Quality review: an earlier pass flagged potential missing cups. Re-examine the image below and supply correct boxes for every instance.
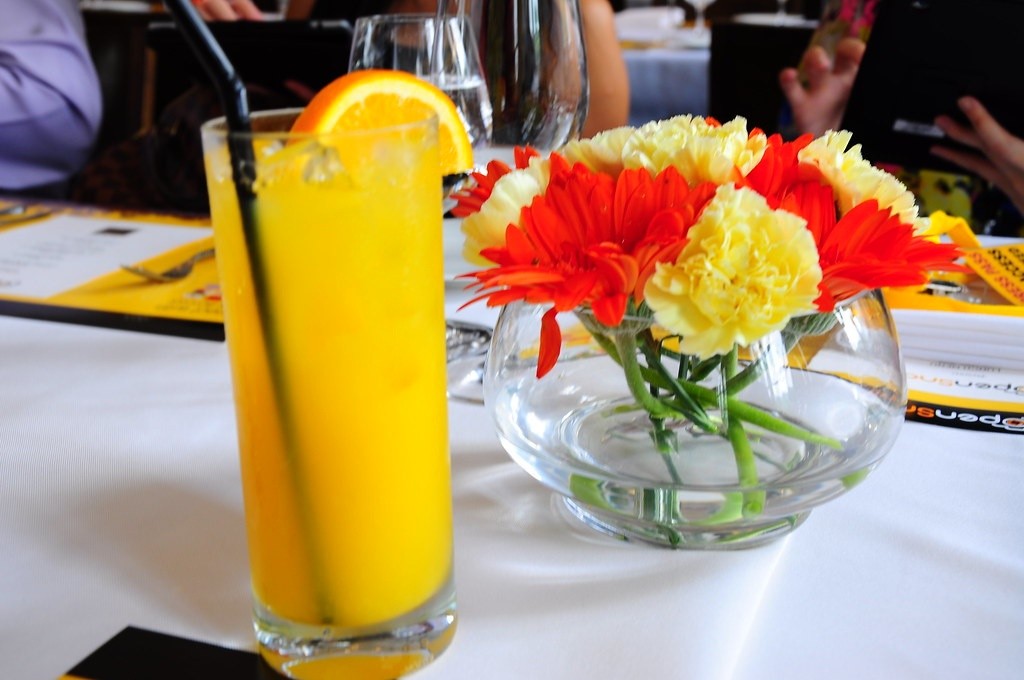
[428,0,588,179]
[346,14,493,181]
[202,107,456,680]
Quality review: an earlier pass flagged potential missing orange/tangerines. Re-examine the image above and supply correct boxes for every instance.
[283,70,474,178]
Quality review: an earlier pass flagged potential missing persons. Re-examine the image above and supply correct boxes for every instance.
[706,0,1024,238]
[191,1,632,146]
[0,0,103,203]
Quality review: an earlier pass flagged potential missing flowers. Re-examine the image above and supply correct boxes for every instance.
[449,111,980,542]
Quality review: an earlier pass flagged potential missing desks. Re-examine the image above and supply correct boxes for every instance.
[0,190,1021,679]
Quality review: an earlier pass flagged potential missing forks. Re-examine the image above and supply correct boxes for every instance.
[121,247,215,282]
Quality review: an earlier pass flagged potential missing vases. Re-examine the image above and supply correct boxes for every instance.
[481,284,907,550]
